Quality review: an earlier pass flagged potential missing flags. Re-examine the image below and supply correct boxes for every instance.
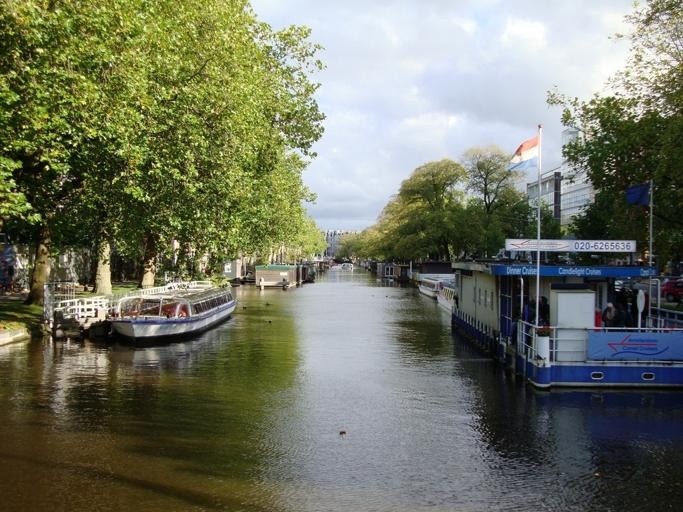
[508,133,540,163]
[625,182,652,209]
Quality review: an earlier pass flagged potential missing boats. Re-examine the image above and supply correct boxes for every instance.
[108,283,240,343]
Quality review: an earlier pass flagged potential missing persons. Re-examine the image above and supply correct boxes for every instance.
[0,250,9,293]
[524,283,649,336]
[4,247,17,292]
